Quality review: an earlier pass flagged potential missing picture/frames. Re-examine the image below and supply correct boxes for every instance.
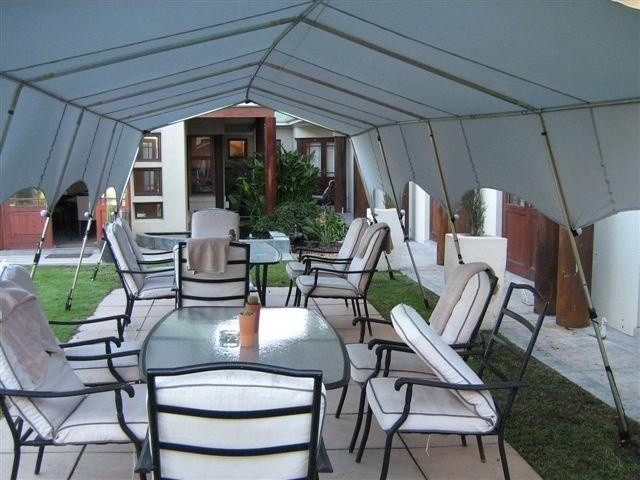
[228,139,247,158]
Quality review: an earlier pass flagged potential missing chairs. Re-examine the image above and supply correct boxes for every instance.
[311,179,335,210]
[133,358,336,479]
[189,208,241,239]
[167,238,250,306]
[0,262,151,480]
[296,221,394,337]
[284,216,373,308]
[101,216,175,325]
[355,279,549,479]
[332,260,499,454]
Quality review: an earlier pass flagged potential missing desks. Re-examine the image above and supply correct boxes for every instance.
[172,237,283,307]
[137,305,353,392]
